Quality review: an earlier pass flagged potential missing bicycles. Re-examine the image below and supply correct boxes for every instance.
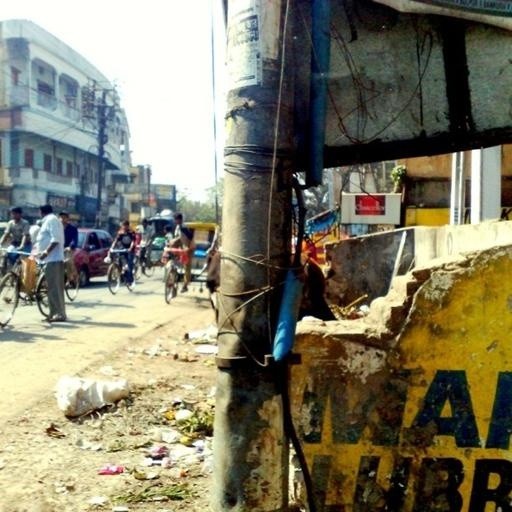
[0,247,80,329]
[108,246,154,293]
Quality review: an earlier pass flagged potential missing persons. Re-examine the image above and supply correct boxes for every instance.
[133,224,142,258]
[28,219,42,244]
[165,212,197,292]
[161,224,174,265]
[28,204,68,323]
[135,219,156,273]
[0,205,32,276]
[109,219,136,287]
[57,210,79,288]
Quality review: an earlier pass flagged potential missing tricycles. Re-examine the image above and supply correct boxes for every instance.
[138,215,176,266]
[160,222,220,302]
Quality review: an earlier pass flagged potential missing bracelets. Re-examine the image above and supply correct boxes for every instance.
[20,245,25,248]
[43,249,49,258]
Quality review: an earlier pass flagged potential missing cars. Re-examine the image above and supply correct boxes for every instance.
[40,228,113,288]
